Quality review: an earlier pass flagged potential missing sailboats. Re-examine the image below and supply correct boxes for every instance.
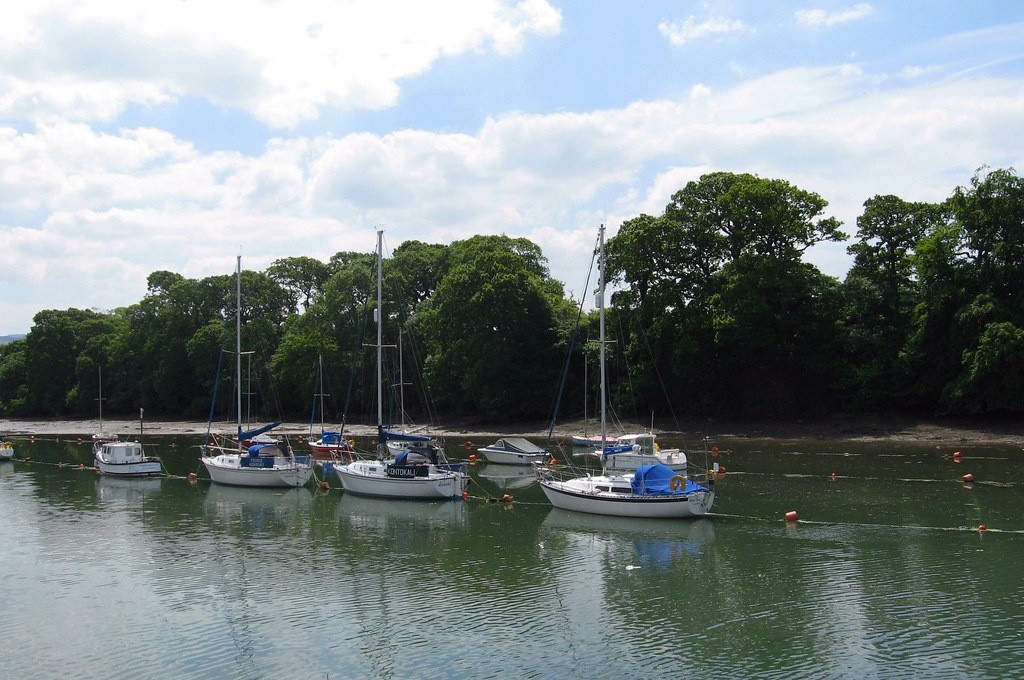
[200,256,314,488]
[329,229,471,501]
[385,326,446,461]
[531,223,725,516]
[307,353,355,460]
[571,349,658,447]
[91,366,119,444]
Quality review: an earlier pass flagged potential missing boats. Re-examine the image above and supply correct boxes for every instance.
[594,412,689,475]
[476,437,553,465]
[0,440,14,460]
[93,439,164,476]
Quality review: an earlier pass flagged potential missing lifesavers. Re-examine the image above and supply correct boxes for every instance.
[4,442,12,450]
[670,474,687,491]
[346,439,357,451]
